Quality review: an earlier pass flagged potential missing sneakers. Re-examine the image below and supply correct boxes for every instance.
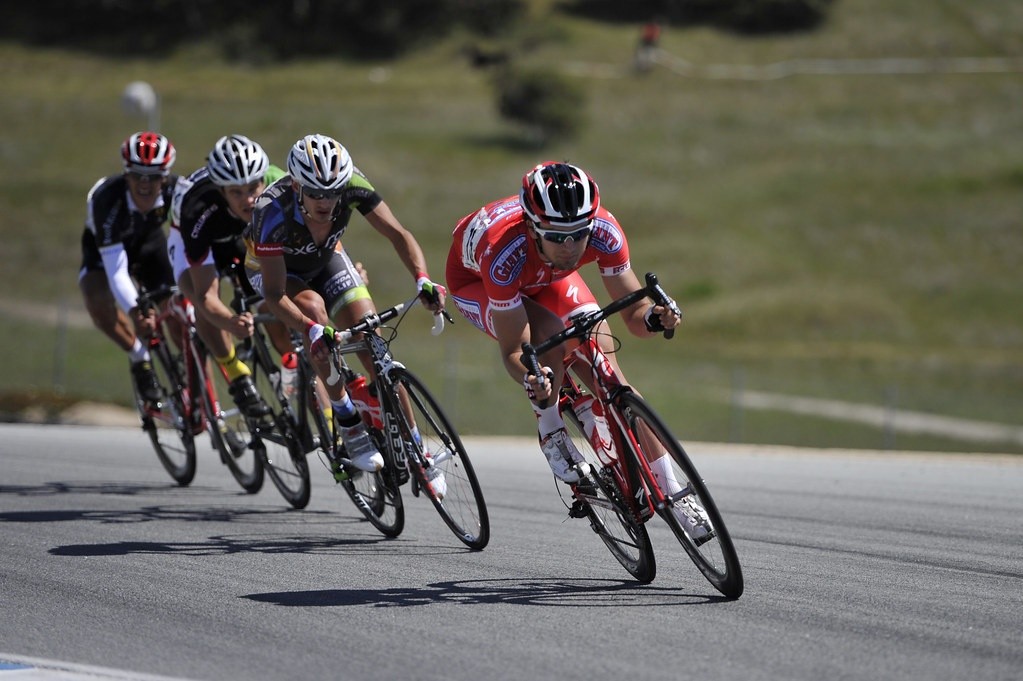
[538,428,591,482]
[415,448,447,498]
[228,375,270,417]
[221,424,243,458]
[128,350,163,401]
[673,495,714,539]
[334,416,384,472]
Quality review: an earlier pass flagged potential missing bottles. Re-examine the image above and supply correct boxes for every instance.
[571,393,617,464]
[368,380,382,430]
[268,365,290,407]
[281,352,297,399]
[591,399,618,462]
[345,368,371,424]
[175,354,188,388]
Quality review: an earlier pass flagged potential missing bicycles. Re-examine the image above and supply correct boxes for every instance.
[228,290,385,518]
[519,271,744,601]
[314,282,490,550]
[126,283,265,493]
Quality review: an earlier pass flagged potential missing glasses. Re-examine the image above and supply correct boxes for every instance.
[220,185,265,200]
[130,172,163,184]
[531,219,594,244]
[302,185,344,200]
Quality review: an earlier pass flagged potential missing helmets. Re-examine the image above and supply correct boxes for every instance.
[519,161,600,226]
[208,134,270,186]
[287,134,353,190]
[121,131,176,176]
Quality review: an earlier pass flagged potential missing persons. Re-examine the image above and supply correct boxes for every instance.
[446,162,717,547]
[244,133,447,499]
[79,130,370,463]
